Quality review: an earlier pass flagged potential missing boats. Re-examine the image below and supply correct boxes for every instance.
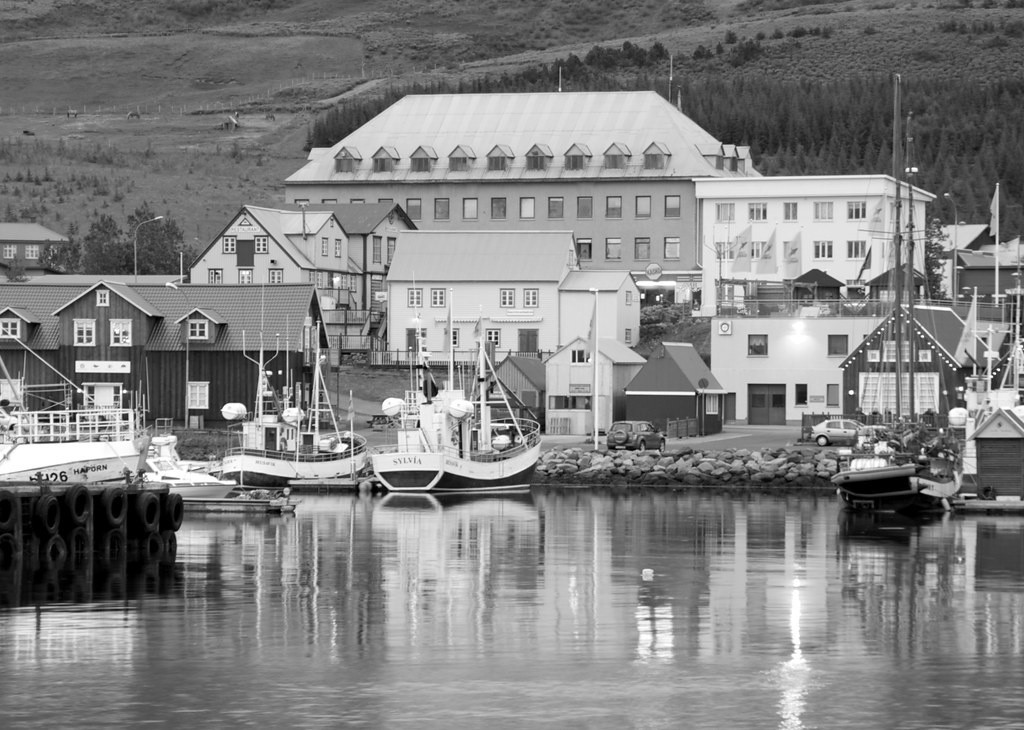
[221,288,368,489]
[141,445,238,504]
[361,289,545,491]
[1,324,144,499]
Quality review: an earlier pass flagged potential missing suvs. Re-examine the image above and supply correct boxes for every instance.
[608,422,670,451]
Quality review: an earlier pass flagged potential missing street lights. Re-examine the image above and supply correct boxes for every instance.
[133,214,165,280]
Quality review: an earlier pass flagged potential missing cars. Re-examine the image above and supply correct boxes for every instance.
[812,419,888,447]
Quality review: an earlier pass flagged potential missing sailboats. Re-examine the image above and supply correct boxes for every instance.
[830,68,965,522]
